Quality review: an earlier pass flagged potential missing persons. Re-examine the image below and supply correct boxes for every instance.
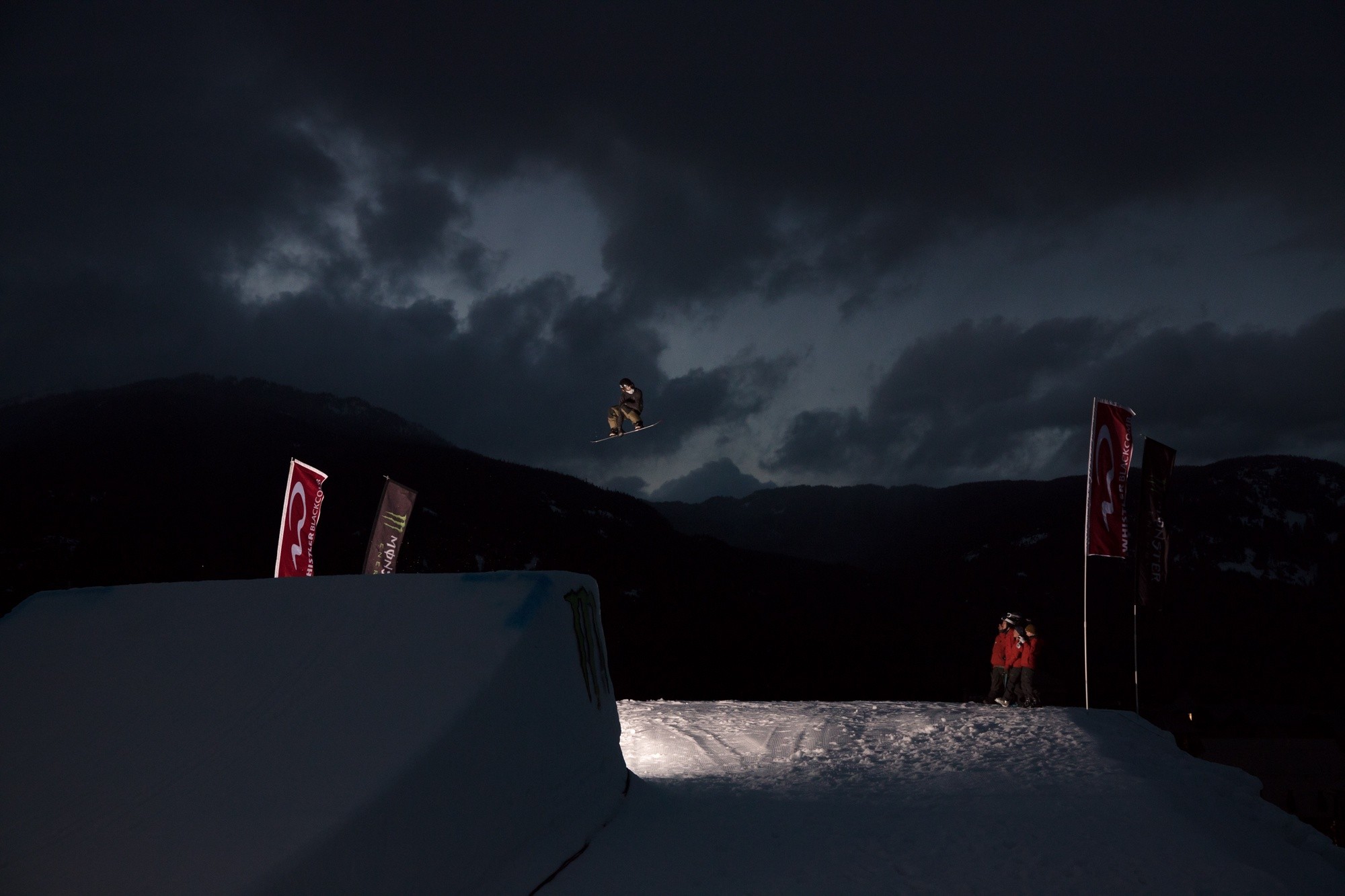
[982,613,1043,708]
[607,378,644,436]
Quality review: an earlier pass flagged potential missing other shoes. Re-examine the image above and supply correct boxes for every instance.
[1028,698,1046,709]
[1023,699,1032,708]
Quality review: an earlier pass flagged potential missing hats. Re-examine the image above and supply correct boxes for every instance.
[1015,625,1024,636]
[1025,624,1037,634]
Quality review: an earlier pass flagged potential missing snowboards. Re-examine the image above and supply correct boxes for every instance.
[590,419,663,444]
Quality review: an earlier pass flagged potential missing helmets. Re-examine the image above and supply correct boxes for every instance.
[1001,613,1021,625]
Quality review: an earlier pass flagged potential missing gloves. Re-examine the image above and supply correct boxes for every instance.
[617,427,625,437]
[618,403,625,411]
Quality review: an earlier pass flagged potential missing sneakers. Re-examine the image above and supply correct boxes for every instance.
[983,697,997,705]
[995,698,1009,707]
[635,421,643,429]
[609,428,618,436]
